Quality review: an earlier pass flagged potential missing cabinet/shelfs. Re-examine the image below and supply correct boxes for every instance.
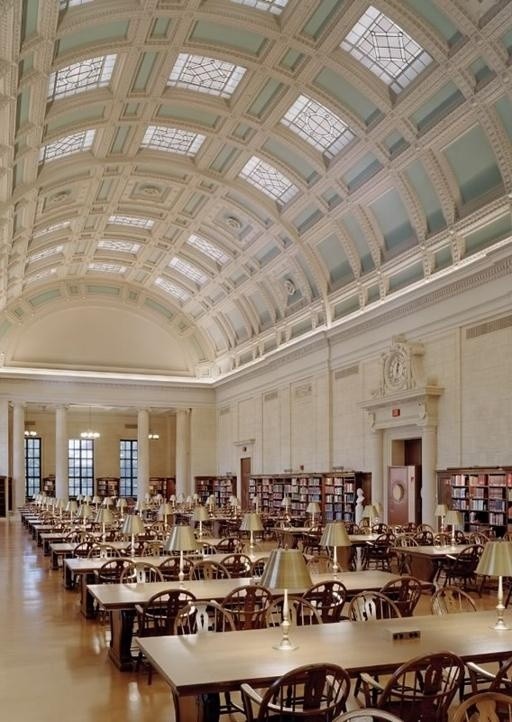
[98,471,370,525]
[436,465,512,536]
[42,474,55,498]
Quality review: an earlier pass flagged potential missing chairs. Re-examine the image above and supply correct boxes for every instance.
[18,494,512,722]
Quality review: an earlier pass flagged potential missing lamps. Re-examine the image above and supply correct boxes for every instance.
[148,414,160,440]
[23,406,36,437]
[79,406,101,439]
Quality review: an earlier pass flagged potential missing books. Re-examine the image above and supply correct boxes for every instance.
[195,478,354,521]
[44,479,55,496]
[451,474,512,526]
[98,480,118,496]
[149,480,167,497]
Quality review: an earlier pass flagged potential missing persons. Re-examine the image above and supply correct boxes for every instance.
[355,488,365,524]
